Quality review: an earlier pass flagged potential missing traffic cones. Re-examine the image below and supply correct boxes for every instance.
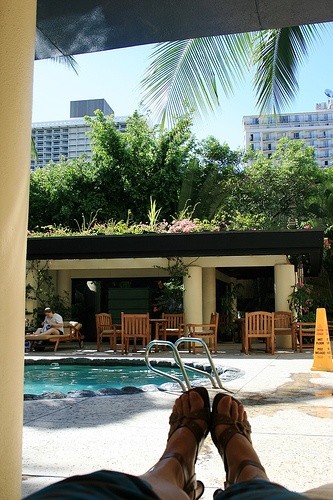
[310,307,333,372]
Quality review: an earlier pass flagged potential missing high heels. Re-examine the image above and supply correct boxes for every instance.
[210,393,265,500]
[160,387,210,500]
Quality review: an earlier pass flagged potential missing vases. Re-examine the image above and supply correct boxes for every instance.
[221,330,231,343]
[297,327,304,344]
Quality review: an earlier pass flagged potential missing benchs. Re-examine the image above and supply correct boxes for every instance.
[296,320,333,352]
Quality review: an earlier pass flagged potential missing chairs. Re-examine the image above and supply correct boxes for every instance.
[122,311,152,355]
[273,311,296,350]
[25,321,85,351]
[187,312,220,355]
[95,313,122,354]
[156,312,187,350]
[245,311,275,354]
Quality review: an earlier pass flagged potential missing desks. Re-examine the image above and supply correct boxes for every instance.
[148,319,175,352]
[232,318,285,352]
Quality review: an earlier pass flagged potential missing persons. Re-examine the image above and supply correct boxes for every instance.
[33,306,65,335]
[22,386,312,499]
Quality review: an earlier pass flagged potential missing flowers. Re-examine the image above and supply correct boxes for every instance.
[221,283,244,332]
[153,256,191,313]
[287,280,314,322]
